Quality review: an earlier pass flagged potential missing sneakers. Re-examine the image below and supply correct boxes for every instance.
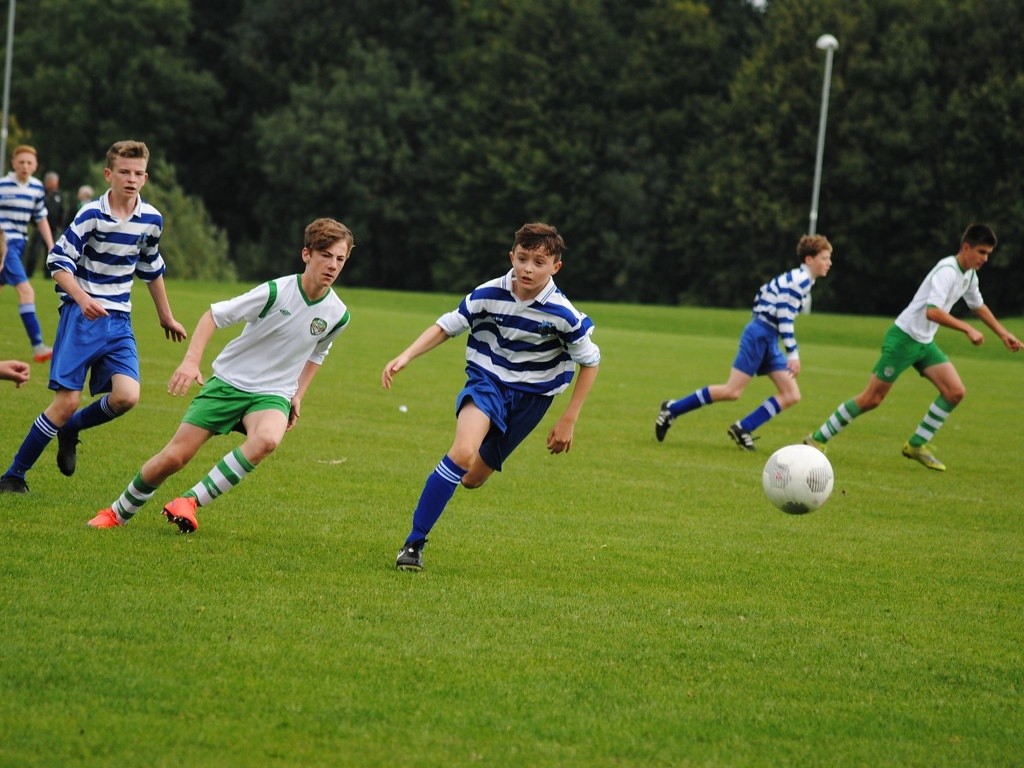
[728,421,757,454]
[394,538,428,572]
[654,398,678,442]
[86,505,123,531]
[57,426,80,477]
[901,439,948,472]
[805,433,828,455]
[158,495,199,534]
[33,345,54,364]
[0,474,30,495]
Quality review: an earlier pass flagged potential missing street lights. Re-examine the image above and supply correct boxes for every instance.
[802,34,840,315]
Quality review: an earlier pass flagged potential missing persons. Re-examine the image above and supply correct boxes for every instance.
[0,139,188,496]
[84,218,357,535]
[66,185,94,230]
[23,171,66,278]
[802,219,1024,471]
[380,219,603,573]
[0,145,54,363]
[0,360,31,389]
[653,234,832,451]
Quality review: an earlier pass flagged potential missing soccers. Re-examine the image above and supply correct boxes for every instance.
[762,443,834,516]
